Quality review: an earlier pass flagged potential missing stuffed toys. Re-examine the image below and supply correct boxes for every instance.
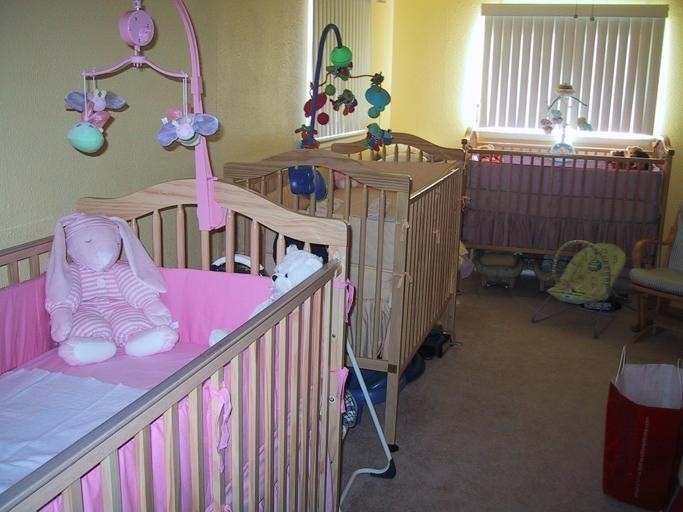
[476,144,504,163]
[63,89,125,112]
[43,212,181,365]
[610,151,624,168]
[67,102,110,154]
[626,146,649,169]
[156,106,218,148]
[209,245,322,347]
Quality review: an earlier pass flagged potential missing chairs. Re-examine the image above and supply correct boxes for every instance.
[530,238,628,340]
[625,203,683,348]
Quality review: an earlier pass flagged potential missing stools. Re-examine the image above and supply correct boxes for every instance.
[475,254,522,289]
[533,259,564,295]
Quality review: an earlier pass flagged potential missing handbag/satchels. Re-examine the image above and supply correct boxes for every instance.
[602,347,683,509]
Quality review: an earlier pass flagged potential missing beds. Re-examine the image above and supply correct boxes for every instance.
[0,176,350,512]
[218,126,466,452]
[458,127,673,297]
